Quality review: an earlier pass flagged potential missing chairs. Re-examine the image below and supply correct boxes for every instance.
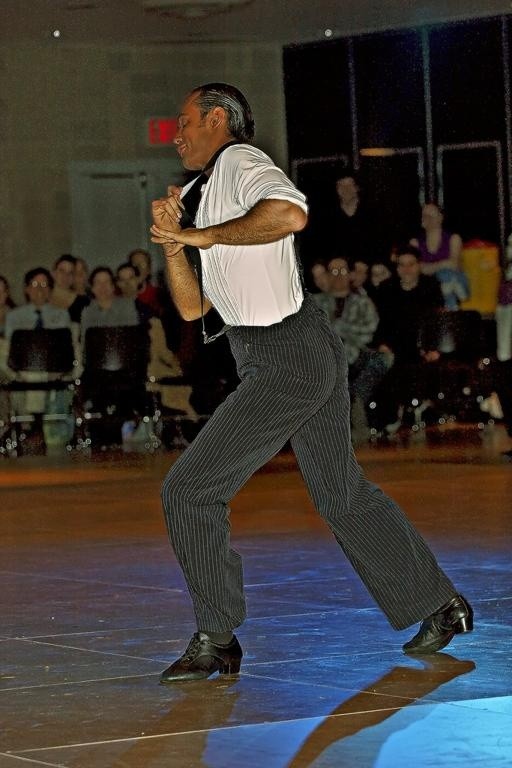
[0,309,492,457]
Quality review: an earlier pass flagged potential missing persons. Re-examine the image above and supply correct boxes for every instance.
[308,247,512,445]
[314,173,384,264]
[0,248,241,461]
[403,203,473,312]
[144,78,478,687]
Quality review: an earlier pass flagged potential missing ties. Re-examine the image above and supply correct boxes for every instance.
[34,309,44,329]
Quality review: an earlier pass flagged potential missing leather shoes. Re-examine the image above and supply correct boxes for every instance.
[402,593,475,655]
[159,631,242,682]
